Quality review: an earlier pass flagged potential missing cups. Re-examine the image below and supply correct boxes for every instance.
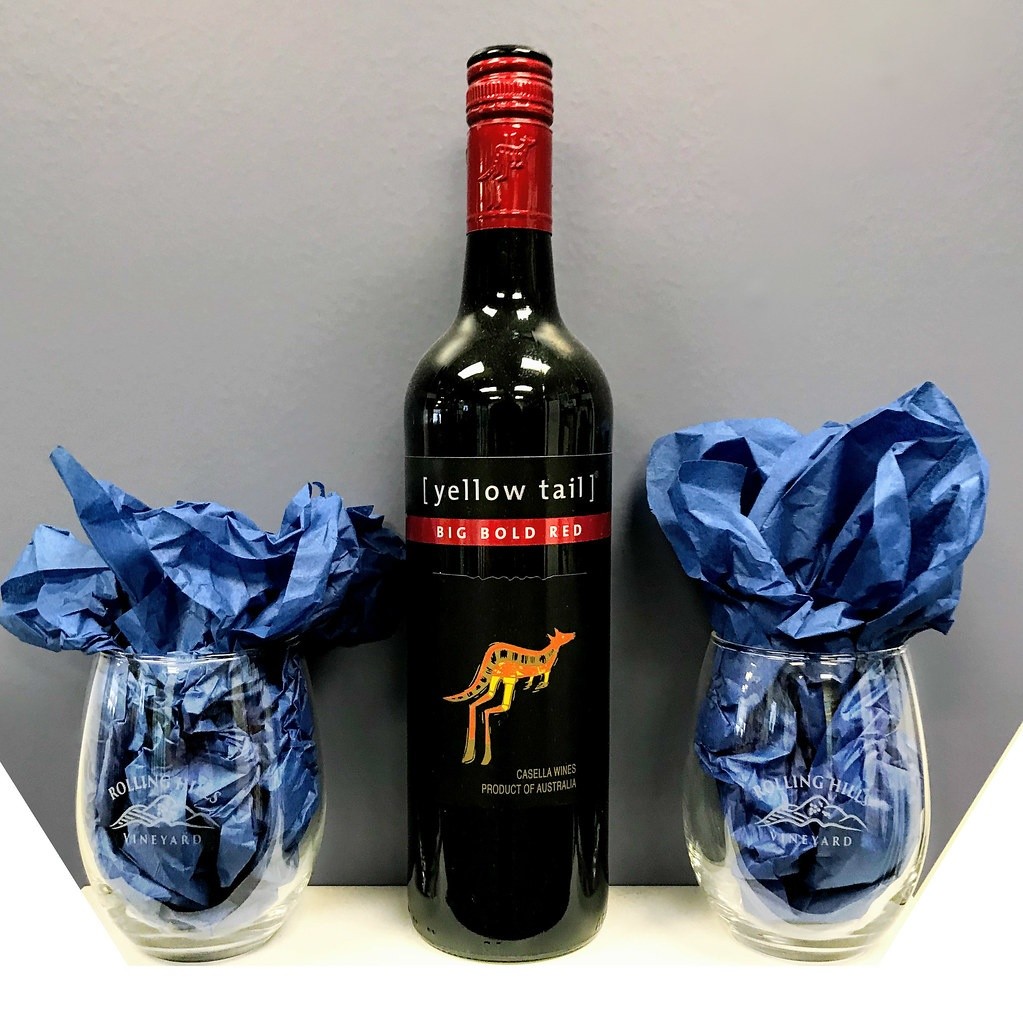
[75,633,328,963]
[681,631,931,961]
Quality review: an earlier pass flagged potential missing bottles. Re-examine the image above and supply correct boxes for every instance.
[404,46,613,964]
